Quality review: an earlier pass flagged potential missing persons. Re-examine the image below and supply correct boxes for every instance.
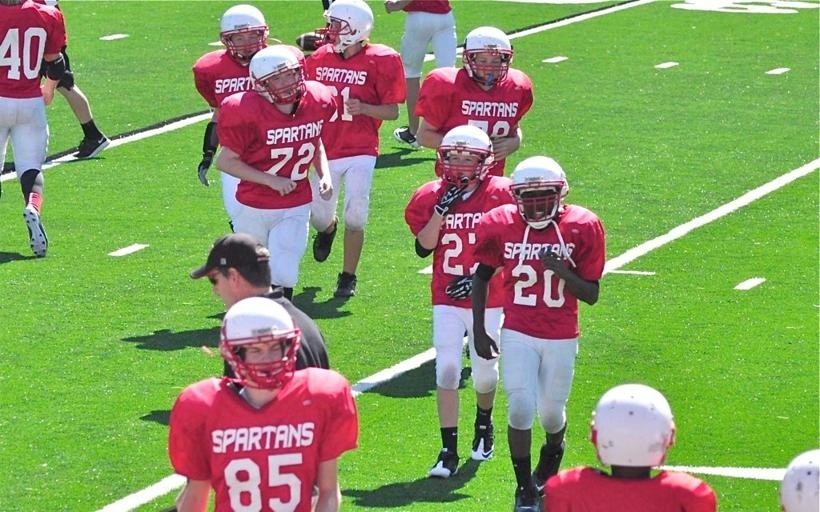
[416,26,534,177]
[322,0,336,27]
[32,0,110,160]
[0,0,67,257]
[169,296,359,512]
[192,4,269,237]
[542,384,716,512]
[306,0,406,298]
[472,156,605,512]
[405,125,517,479]
[190,233,329,380]
[215,45,333,302]
[384,0,457,149]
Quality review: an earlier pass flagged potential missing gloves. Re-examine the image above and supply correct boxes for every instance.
[434,177,469,215]
[443,274,475,301]
[197,149,213,187]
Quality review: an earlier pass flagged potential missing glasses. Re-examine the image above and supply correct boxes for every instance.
[210,269,224,284]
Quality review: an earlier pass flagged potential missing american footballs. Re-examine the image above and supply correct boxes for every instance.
[295,32,326,51]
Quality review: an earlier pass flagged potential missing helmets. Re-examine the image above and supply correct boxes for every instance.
[220,296,300,389]
[508,156,569,231]
[592,384,674,469]
[220,5,269,59]
[323,1,374,54]
[462,27,514,85]
[249,44,307,105]
[435,125,495,184]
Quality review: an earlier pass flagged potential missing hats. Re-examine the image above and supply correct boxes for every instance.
[189,233,269,279]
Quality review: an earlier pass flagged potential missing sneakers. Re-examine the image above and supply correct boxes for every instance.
[73,133,111,159]
[313,216,339,262]
[471,419,495,460]
[332,271,357,298]
[428,450,460,478]
[23,205,48,260]
[513,485,542,512]
[532,439,565,496]
[393,127,422,149]
